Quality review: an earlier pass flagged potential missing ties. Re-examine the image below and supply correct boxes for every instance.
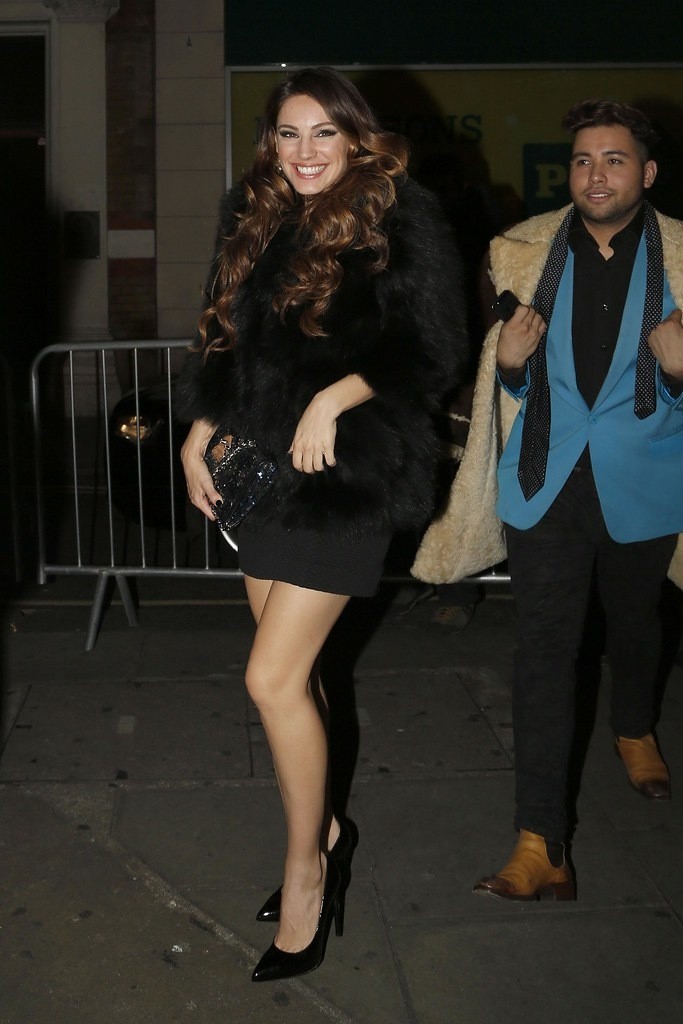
[517,196,664,503]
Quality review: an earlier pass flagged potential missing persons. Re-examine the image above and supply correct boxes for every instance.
[167,67,467,982]
[467,96,682,904]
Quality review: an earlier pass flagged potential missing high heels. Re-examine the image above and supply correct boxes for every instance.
[251,853,345,982]
[255,818,352,923]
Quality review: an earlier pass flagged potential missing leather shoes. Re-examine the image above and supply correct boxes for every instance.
[472,828,577,903]
[614,733,672,801]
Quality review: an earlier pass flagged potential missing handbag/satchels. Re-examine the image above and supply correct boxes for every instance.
[203,433,281,532]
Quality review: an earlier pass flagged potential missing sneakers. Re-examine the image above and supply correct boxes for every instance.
[385,580,437,617]
[427,603,477,634]
